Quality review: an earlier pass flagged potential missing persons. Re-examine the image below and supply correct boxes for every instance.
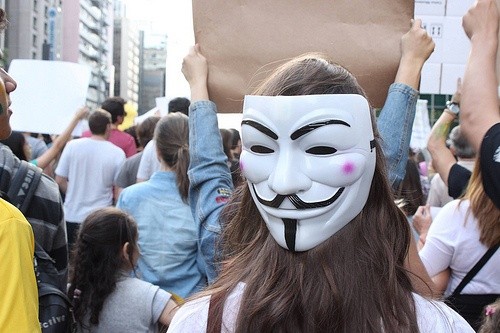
[0,0,500,332]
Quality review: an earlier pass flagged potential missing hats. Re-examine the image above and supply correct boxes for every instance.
[479,123,500,210]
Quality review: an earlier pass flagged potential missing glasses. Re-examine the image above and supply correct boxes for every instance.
[116,111,127,117]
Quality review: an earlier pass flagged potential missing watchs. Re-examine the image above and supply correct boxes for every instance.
[444,101,460,119]
